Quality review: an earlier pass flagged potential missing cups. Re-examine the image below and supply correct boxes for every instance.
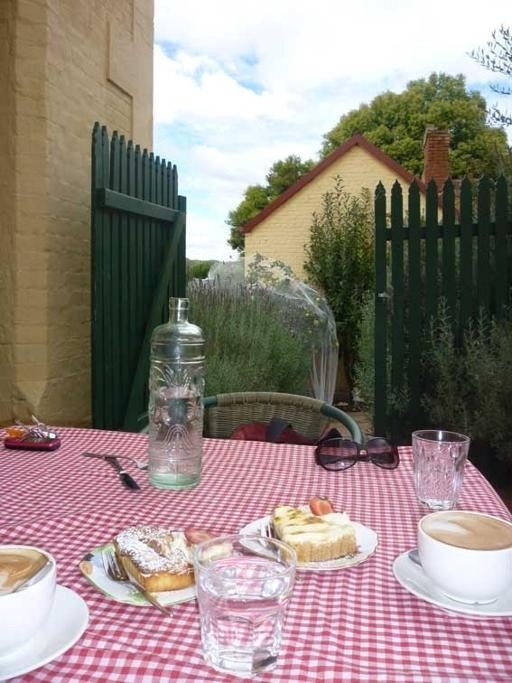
[410,429,469,512]
[0,545,55,649]
[417,511,512,609]
[193,533,294,678]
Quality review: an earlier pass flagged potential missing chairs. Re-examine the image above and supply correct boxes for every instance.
[138,390,364,445]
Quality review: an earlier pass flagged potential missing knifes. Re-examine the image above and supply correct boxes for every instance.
[105,458,144,495]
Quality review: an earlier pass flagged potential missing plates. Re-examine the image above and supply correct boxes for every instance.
[236,512,381,573]
[79,535,199,611]
[0,583,89,681]
[393,545,512,619]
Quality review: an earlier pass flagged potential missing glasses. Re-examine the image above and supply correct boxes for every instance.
[315,437,399,470]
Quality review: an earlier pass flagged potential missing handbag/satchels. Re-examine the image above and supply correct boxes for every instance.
[230,417,341,447]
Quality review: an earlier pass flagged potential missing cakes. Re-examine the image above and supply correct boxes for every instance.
[110,521,231,594]
[259,495,357,564]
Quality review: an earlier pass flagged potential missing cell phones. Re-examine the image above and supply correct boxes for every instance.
[4,437,60,450]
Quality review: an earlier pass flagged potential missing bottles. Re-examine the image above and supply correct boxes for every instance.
[149,294,205,488]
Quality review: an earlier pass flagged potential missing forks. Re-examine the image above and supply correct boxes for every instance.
[97,544,169,617]
[81,451,150,472]
